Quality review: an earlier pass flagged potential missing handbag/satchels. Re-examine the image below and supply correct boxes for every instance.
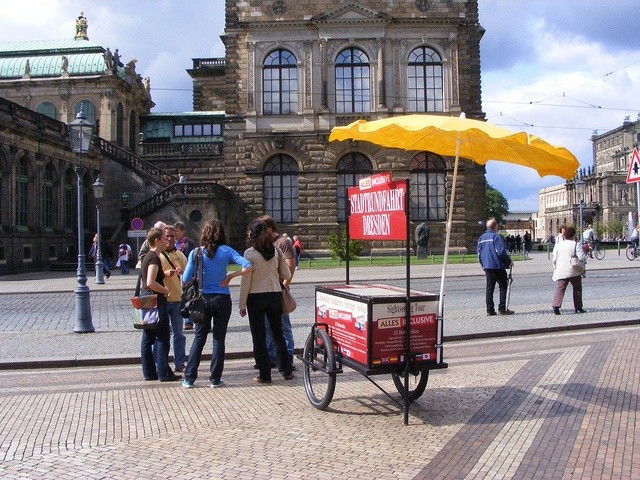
[571,242,586,273]
[131,264,161,330]
[276,248,296,314]
[178,248,199,318]
[185,247,210,323]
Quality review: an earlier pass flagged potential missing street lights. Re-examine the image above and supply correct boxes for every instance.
[529,217,533,240]
[517,219,520,234]
[91,174,104,283]
[65,103,96,333]
[575,178,586,243]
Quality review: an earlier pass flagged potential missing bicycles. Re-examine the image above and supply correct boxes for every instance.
[579,240,606,260]
[626,242,640,261]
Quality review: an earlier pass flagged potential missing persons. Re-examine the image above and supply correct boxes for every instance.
[160,225,187,372]
[183,219,252,389]
[506,231,532,252]
[239,219,293,383]
[102,47,112,69]
[118,242,131,274]
[477,218,515,316]
[552,227,586,315]
[583,224,595,258]
[173,221,196,331]
[113,49,121,72]
[136,221,167,295]
[89,233,111,279]
[140,228,182,382]
[554,226,567,243]
[283,232,292,244]
[254,215,297,371]
[631,225,639,256]
[293,235,301,270]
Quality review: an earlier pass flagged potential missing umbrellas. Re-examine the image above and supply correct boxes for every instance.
[329,112,581,364]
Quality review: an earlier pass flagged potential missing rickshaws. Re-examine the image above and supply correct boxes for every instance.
[296,170,448,409]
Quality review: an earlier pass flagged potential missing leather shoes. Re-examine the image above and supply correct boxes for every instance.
[498,309,514,316]
[486,312,497,316]
[253,362,277,369]
[288,355,296,371]
[158,372,182,382]
[184,323,194,329]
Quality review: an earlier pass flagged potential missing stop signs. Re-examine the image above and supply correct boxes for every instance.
[132,218,143,230]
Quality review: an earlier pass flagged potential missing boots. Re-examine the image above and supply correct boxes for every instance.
[553,306,561,316]
[575,307,587,313]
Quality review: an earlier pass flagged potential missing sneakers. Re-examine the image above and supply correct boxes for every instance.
[253,375,272,384]
[283,373,295,380]
[210,379,225,388]
[175,366,187,373]
[181,379,194,388]
